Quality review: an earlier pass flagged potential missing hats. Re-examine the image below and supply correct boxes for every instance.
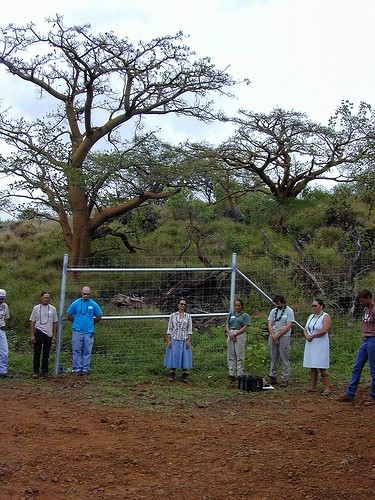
[0,289,6,299]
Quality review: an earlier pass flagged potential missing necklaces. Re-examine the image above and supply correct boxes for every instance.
[364,303,375,320]
[81,298,89,315]
[308,312,323,334]
[234,312,243,317]
[274,305,286,321]
[40,304,49,325]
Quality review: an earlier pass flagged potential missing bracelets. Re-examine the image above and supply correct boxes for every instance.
[228,332,231,334]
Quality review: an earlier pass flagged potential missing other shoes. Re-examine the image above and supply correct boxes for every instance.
[280,381,288,386]
[266,376,276,384]
[82,369,91,375]
[321,388,330,395]
[167,370,176,381]
[44,373,52,378]
[308,386,317,391]
[33,373,39,378]
[336,395,353,401]
[74,369,82,375]
[182,371,189,383]
[1,373,13,379]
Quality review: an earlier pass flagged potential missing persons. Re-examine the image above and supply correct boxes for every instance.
[163,299,194,382]
[337,288,375,402]
[226,299,251,380]
[66,286,103,376]
[0,289,10,377]
[266,295,294,387]
[29,292,58,379]
[303,299,331,395]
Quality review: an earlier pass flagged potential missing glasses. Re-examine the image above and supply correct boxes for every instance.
[311,305,317,308]
[42,297,49,299]
[82,292,89,296]
[180,303,186,306]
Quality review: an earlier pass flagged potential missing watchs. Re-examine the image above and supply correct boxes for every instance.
[234,332,237,336]
